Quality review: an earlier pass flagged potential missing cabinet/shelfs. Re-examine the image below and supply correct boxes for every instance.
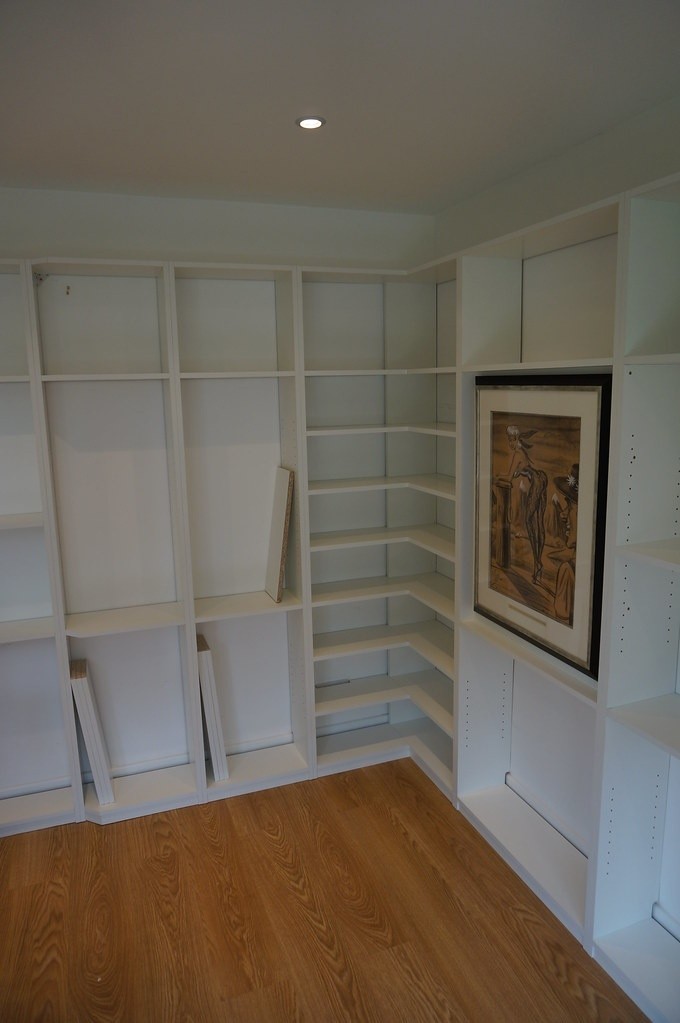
[0,168,680,1023]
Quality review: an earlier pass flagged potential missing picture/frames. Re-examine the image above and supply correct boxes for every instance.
[472,371,614,681]
